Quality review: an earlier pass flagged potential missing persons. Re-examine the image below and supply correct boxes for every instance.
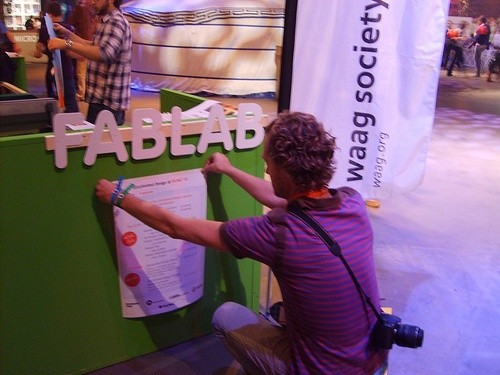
[96,112,393,375]
[441,15,500,83]
[0,0,133,126]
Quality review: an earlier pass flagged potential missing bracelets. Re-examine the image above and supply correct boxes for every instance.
[64,30,71,38]
[65,39,73,50]
[110,176,123,205]
[116,183,135,207]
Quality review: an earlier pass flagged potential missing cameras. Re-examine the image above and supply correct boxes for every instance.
[371,313,424,349]
